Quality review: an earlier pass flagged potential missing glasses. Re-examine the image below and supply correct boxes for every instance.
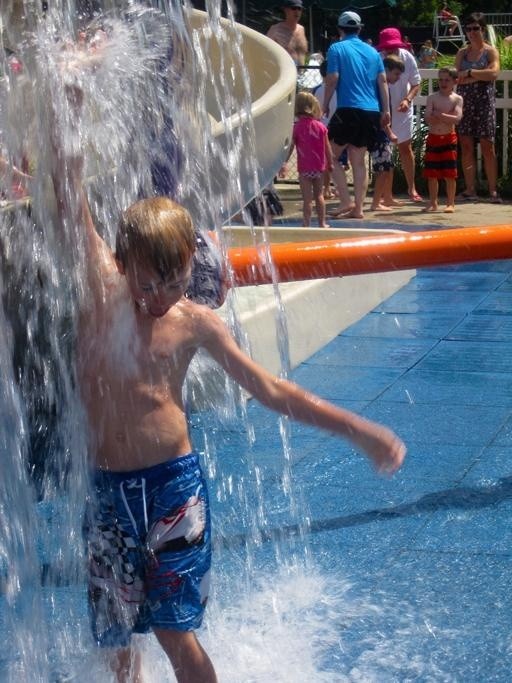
[465,26,480,32]
[291,7,301,10]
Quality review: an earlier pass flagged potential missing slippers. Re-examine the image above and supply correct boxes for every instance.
[455,192,479,201]
[407,193,423,202]
[487,196,503,203]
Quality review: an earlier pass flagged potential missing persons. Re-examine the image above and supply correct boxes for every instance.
[439,2,459,35]
[419,40,441,70]
[420,68,464,214]
[37,60,409,682]
[455,12,503,204]
[266,2,424,228]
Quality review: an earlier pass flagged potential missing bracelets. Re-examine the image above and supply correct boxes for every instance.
[468,68,472,78]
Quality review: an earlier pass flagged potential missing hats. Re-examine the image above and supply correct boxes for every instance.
[338,12,362,27]
[374,28,412,49]
[286,0,303,7]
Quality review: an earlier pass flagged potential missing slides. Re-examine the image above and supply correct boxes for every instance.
[0,0,296,262]
